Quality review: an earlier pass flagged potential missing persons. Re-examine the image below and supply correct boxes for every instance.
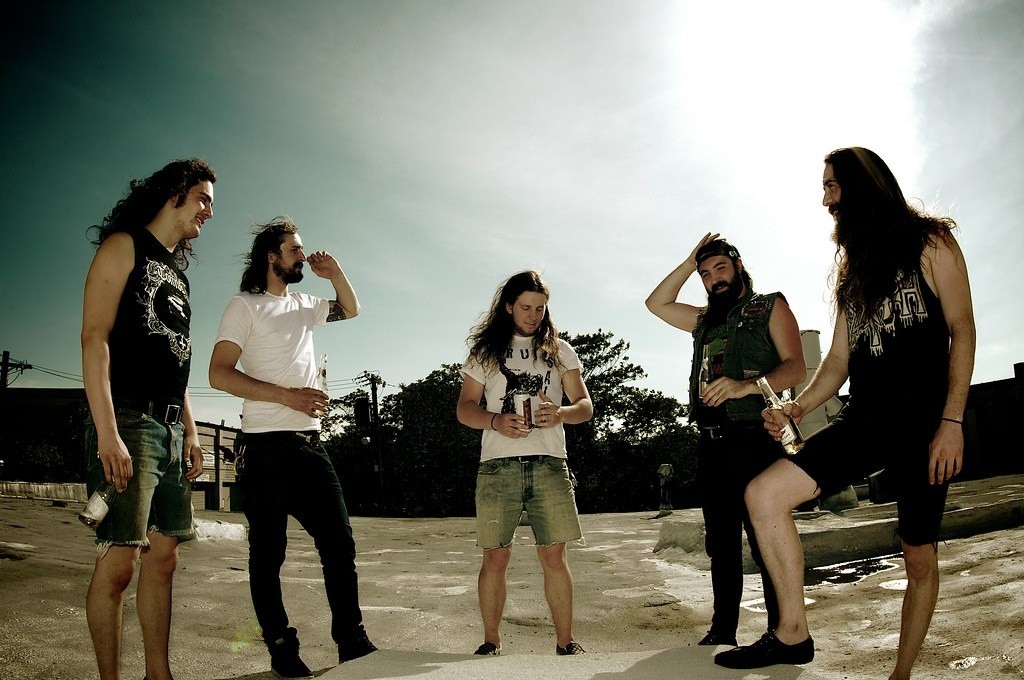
[644,233,807,647]
[209,215,379,680]
[713,147,976,680]
[454,271,595,655]
[81,156,216,680]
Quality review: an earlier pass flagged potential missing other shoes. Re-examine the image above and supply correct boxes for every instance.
[700,633,738,647]
[556,641,587,654]
[473,643,501,655]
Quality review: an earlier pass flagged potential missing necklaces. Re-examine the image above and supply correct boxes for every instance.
[513,327,527,337]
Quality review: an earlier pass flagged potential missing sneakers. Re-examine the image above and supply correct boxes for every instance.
[713,628,815,669]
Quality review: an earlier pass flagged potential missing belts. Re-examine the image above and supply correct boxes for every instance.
[110,393,184,425]
[701,421,764,440]
[497,454,548,463]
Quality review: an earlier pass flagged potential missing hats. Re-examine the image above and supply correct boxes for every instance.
[696,242,741,269]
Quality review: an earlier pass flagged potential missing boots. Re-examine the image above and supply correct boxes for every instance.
[338,624,378,664]
[262,625,314,680]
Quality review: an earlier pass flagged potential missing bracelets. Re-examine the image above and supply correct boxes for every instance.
[941,417,963,424]
[491,412,499,431]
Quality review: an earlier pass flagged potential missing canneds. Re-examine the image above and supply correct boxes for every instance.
[514,390,533,432]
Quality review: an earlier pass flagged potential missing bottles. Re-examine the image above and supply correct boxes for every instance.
[756,376,805,454]
[78,454,134,531]
[313,353,329,416]
[699,345,713,406]
